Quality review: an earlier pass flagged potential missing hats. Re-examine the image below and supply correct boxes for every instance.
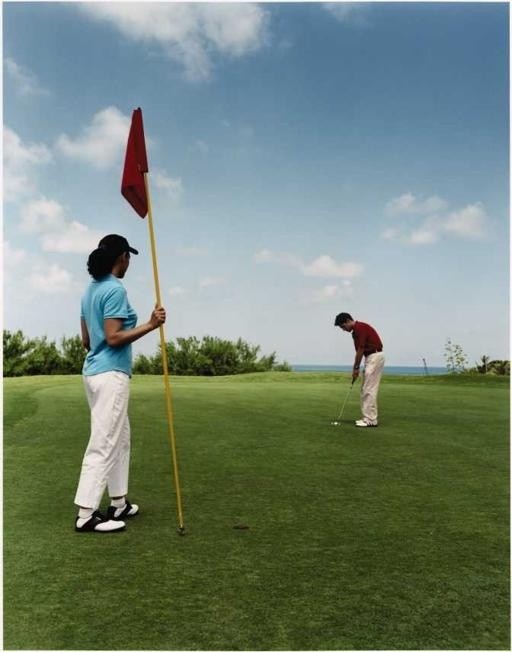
[98,234,138,255]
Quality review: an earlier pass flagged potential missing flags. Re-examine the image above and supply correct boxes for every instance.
[119,107,150,218]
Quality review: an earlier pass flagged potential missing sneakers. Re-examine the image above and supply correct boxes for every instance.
[74,509,126,533]
[105,500,139,521]
[355,417,378,427]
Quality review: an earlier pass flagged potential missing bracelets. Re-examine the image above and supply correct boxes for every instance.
[354,366,359,370]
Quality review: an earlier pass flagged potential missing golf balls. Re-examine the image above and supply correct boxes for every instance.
[334,420,338,425]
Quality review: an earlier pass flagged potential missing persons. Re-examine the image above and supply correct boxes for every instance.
[70,234,167,535]
[334,312,386,427]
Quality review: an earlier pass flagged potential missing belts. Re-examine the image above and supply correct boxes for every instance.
[365,348,382,357]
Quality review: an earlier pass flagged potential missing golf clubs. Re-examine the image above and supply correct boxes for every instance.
[331,379,355,423]
